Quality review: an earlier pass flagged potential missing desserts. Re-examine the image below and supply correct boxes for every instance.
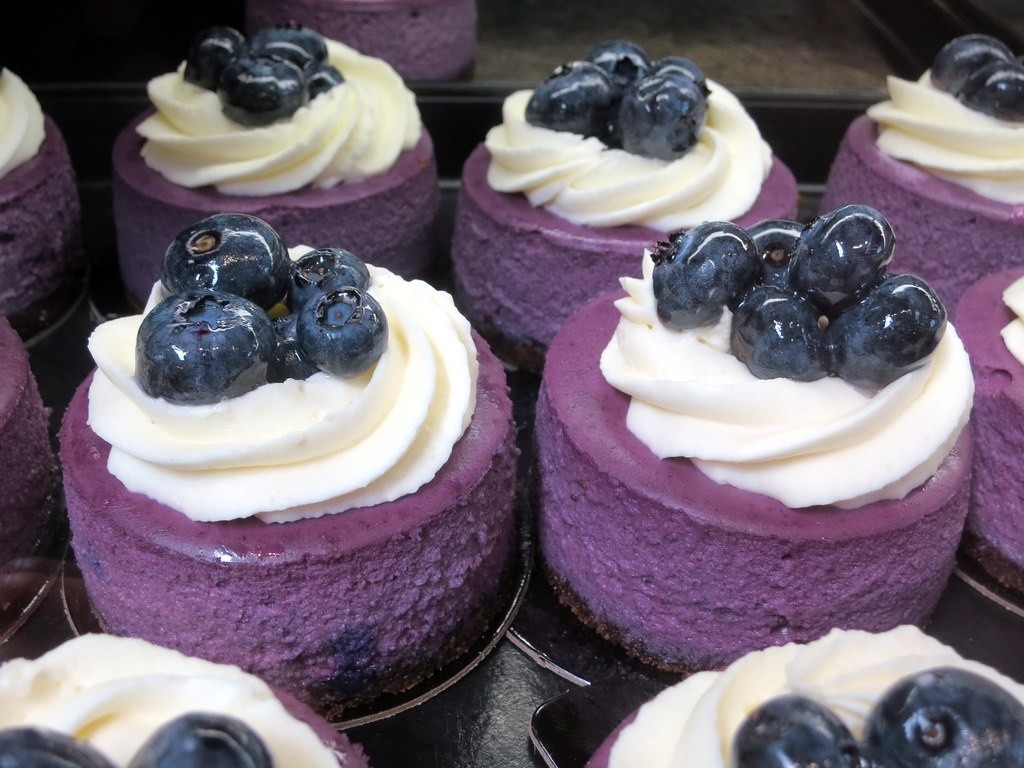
[0,0,1024,768]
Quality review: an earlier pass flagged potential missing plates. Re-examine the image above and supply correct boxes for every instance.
[0,478,68,648]
[945,550,1024,631]
[506,580,641,702]
[528,668,663,768]
[5,248,85,354]
[58,472,534,734]
[80,216,136,327]
